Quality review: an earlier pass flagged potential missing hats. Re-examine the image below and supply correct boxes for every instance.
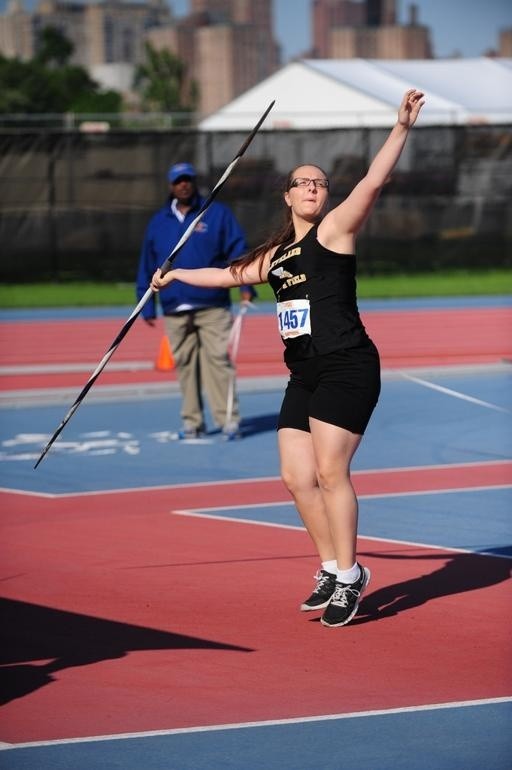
[167,163,197,184]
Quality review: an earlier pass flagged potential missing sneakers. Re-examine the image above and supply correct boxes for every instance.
[223,429,242,442]
[301,568,337,613]
[177,425,207,440]
[320,561,372,628]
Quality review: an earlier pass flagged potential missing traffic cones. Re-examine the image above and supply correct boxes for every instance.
[155,333,176,373]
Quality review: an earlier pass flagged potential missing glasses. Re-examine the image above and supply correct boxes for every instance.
[287,177,330,193]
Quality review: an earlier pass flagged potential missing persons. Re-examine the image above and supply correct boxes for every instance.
[149,88,425,627]
[135,161,258,440]
